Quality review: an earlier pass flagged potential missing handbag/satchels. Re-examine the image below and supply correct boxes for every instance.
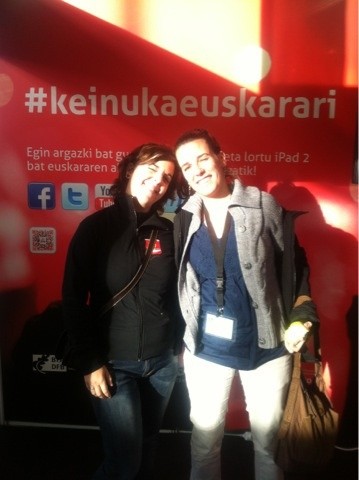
[265,295,341,480]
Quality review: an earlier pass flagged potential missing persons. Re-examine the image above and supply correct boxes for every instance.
[61,143,190,480]
[174,128,319,480]
